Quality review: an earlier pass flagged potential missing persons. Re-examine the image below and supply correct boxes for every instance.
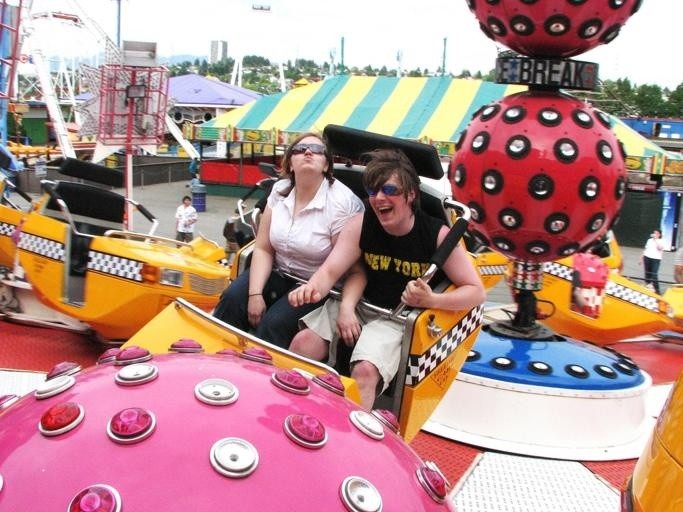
[185,174,200,189]
[212,132,366,349]
[288,149,488,417]
[641,229,665,295]
[188,157,198,177]
[174,195,198,248]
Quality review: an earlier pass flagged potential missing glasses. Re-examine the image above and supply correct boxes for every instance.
[291,143,325,156]
[365,185,399,197]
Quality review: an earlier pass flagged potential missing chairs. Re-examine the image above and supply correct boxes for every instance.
[40,158,159,277]
[229,124,472,346]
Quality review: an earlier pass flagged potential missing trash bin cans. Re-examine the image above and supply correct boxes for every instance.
[191,185,208,212]
